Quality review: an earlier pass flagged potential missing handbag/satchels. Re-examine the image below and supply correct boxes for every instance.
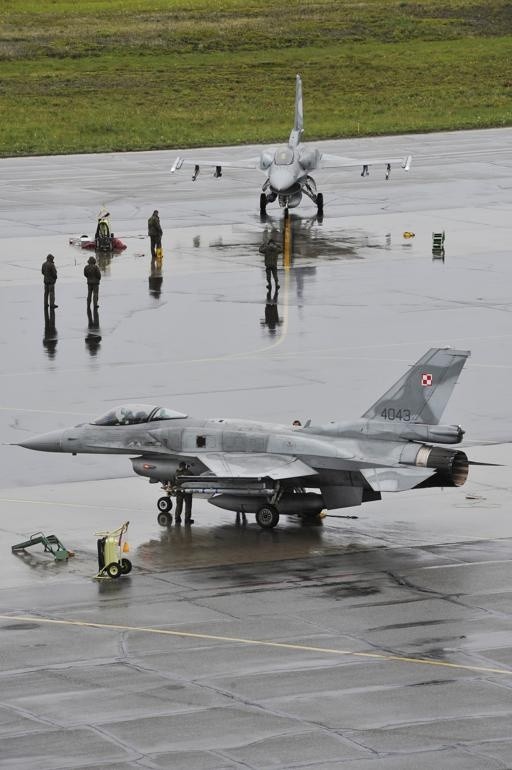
[156,247,163,258]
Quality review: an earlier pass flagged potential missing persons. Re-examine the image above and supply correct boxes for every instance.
[174,494,194,524]
[147,256,163,300]
[260,289,284,337]
[258,239,282,289]
[148,210,163,257]
[84,256,102,311]
[174,523,193,569]
[43,308,58,361]
[41,254,58,309]
[84,309,103,358]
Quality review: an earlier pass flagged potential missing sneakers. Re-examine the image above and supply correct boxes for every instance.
[266,284,281,289]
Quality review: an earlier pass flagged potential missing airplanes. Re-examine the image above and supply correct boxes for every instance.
[164,72,419,223]
[11,337,512,539]
